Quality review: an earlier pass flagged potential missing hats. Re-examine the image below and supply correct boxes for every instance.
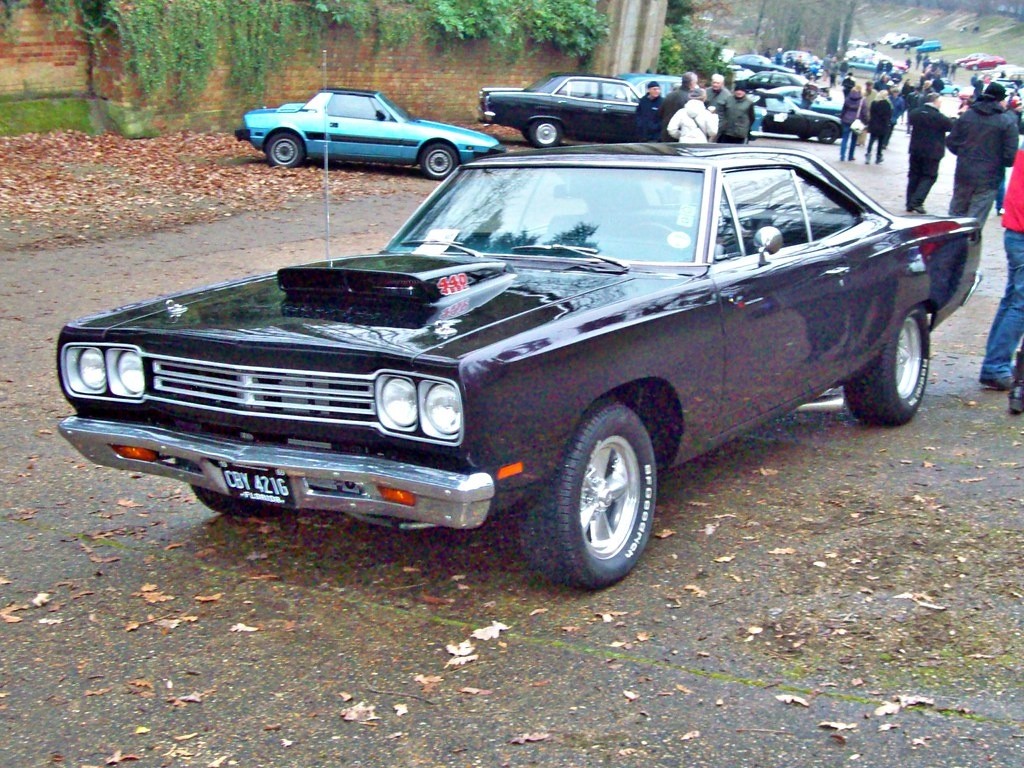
[689,89,706,99]
[734,81,749,93]
[648,81,659,89]
[923,80,932,89]
[984,82,1009,102]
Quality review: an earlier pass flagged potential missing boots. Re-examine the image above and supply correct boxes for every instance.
[865,153,872,163]
[876,154,883,163]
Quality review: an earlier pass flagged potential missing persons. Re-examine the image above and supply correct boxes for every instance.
[978,139,1024,415]
[763,46,856,109]
[907,92,953,213]
[903,45,958,80]
[635,67,755,145]
[945,80,1018,228]
[839,57,945,165]
[958,71,1024,214]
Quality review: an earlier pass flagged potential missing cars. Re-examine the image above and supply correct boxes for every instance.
[476,71,641,149]
[233,88,509,182]
[614,74,701,98]
[54,141,985,593]
[746,93,842,145]
[754,85,843,119]
[727,31,1024,97]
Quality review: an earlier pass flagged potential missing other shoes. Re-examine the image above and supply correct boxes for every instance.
[979,375,1018,390]
[1007,385,1024,412]
[910,206,926,213]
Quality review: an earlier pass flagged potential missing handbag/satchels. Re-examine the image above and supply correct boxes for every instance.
[849,119,867,135]
